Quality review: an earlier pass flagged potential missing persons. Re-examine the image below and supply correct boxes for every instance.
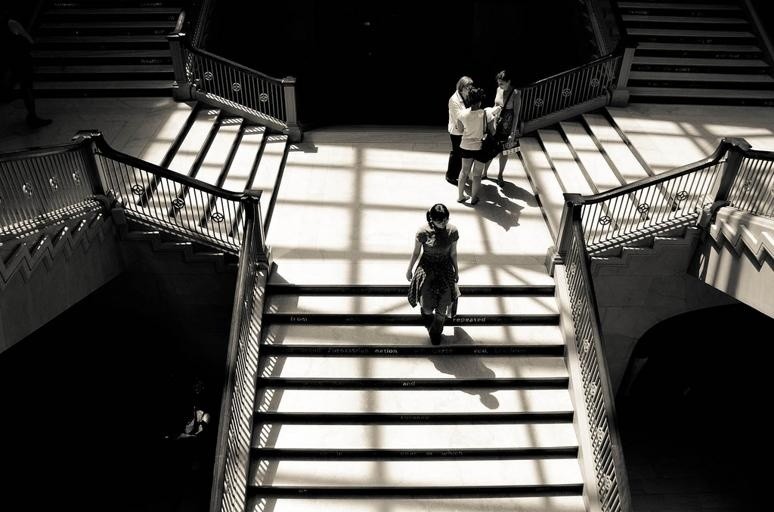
[405,204,461,345]
[456,89,501,205]
[4,15,53,129]
[481,70,520,188]
[445,76,468,185]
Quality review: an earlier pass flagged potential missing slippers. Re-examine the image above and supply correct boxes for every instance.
[471,197,480,205]
[458,197,470,203]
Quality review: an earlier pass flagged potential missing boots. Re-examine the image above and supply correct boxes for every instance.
[421,307,434,331]
[429,318,443,345]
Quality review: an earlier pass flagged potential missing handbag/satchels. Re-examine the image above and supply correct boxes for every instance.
[481,128,502,163]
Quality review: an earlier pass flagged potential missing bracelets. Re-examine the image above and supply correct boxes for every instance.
[510,131,516,136]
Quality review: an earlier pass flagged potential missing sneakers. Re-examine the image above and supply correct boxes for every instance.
[480,175,504,187]
[446,173,458,186]
